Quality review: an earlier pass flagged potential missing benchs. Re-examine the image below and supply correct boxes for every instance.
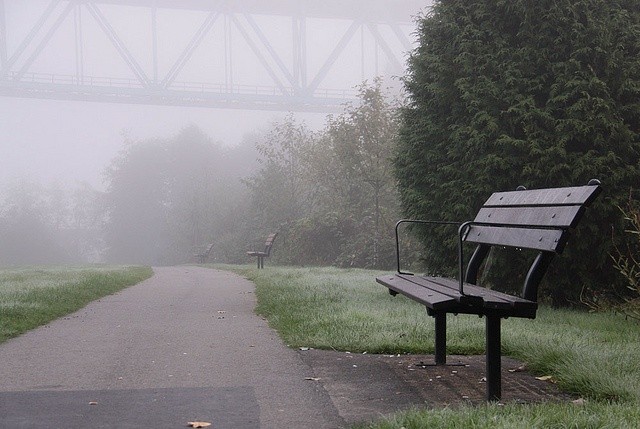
[246,232,279,270]
[373,178,603,404]
[193,241,218,264]
